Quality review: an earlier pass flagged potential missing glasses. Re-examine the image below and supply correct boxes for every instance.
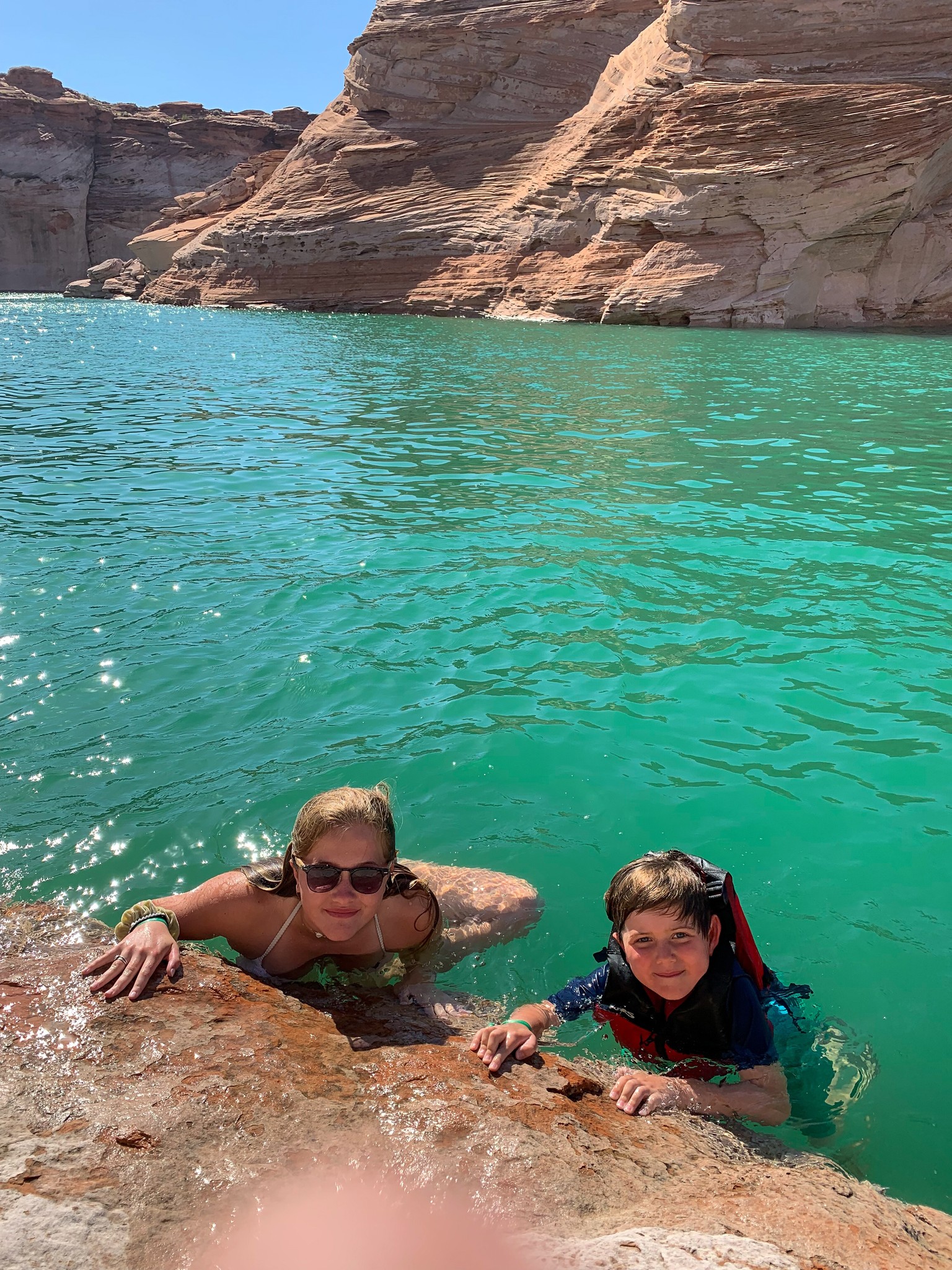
[294,857,393,895]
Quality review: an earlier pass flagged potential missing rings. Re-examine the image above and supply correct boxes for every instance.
[113,954,128,966]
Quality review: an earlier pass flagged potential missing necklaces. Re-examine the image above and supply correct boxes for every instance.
[310,929,326,938]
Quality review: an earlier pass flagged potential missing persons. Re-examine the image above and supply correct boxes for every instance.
[470,847,780,1114]
[81,784,444,1003]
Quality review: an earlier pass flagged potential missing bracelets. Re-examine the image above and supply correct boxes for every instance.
[488,1019,531,1032]
[136,918,168,926]
